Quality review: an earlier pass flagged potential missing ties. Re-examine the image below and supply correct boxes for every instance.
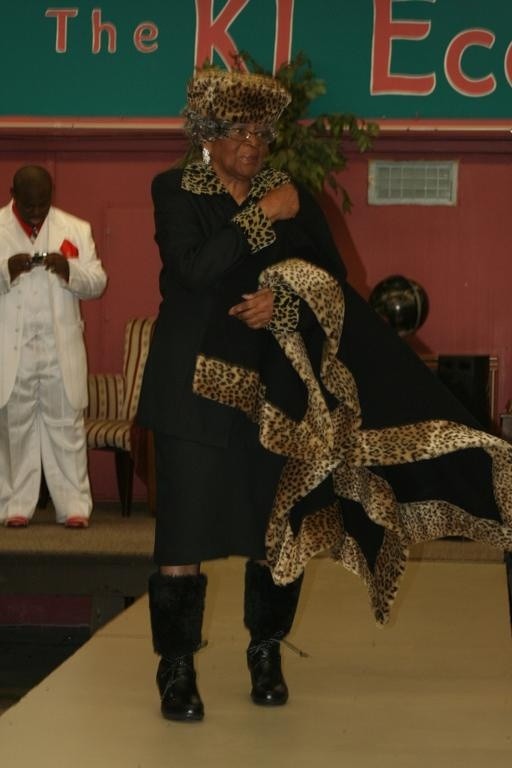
[31,225,38,244]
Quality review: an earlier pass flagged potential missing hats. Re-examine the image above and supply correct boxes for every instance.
[182,72,293,145]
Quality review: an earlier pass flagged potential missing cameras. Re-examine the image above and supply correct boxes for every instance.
[30,254,47,265]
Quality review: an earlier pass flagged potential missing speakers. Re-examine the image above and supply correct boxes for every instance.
[437,354,489,423]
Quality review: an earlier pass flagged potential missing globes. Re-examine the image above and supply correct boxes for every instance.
[369,274,429,340]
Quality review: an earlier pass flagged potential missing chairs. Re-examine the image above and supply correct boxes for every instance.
[84,319,156,519]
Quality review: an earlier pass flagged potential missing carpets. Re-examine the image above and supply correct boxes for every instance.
[0,549,154,717]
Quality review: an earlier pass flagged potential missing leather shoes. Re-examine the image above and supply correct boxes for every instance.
[156,652,204,722]
[247,637,288,706]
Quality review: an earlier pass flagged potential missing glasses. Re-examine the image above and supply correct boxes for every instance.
[228,127,275,146]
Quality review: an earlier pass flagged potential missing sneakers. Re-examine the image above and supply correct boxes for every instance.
[64,517,89,528]
[6,517,31,527]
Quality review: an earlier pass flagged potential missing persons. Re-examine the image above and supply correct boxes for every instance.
[0,166,109,528]
[135,69,346,721]
